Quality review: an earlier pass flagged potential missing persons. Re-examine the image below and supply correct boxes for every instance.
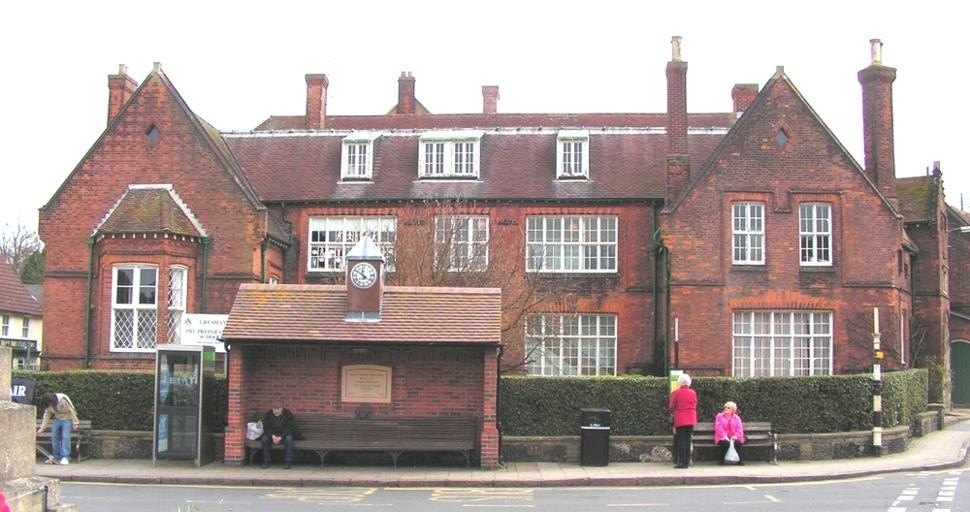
[0,485,10,512]
[667,373,698,468]
[712,400,747,465]
[35,391,81,468]
[255,398,300,469]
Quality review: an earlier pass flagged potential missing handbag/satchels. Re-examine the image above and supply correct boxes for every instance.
[293,431,307,441]
[247,420,264,441]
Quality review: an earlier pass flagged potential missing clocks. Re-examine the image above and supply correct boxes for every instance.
[349,261,378,290]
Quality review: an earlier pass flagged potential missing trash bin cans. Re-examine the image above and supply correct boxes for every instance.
[580,408,611,466]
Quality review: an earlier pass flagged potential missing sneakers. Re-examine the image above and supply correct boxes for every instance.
[283,461,295,468]
[44,455,72,465]
[673,462,689,469]
[719,458,725,465]
[262,460,272,468]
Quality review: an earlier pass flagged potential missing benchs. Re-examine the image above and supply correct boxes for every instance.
[33,417,93,464]
[672,421,780,466]
[246,409,479,470]
